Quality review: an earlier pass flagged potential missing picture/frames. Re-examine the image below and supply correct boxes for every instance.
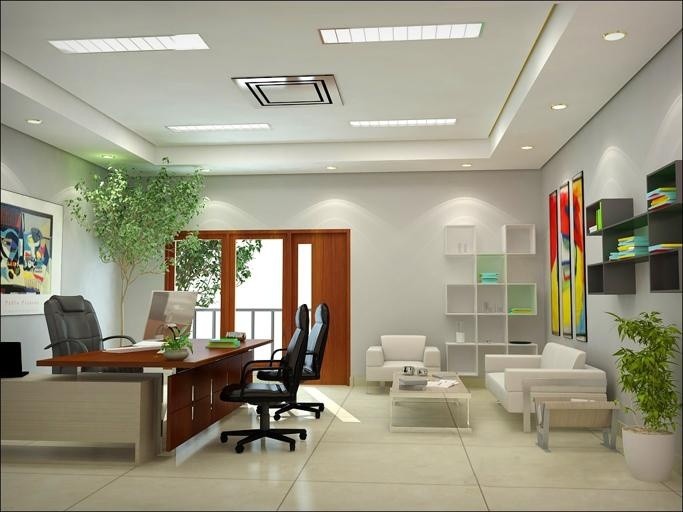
[1,339,274,468]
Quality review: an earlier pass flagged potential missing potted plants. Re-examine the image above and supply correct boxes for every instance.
[0,189,64,316]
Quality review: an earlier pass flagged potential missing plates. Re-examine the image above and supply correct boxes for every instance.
[444,224,538,377]
[586,160,683,295]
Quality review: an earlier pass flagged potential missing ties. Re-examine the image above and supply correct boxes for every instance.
[143,291,198,343]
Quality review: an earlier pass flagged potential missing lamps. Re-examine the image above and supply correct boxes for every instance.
[220,304,309,453]
[256,303,329,421]
[485,342,608,432]
[366,335,441,387]
[44,295,143,374]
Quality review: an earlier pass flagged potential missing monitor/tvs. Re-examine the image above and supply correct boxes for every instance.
[607,233,682,262]
[587,202,604,234]
[203,329,247,350]
[645,187,679,210]
[398,374,427,388]
[425,378,459,389]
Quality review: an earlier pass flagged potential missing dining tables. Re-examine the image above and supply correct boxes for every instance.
[161,326,193,360]
[604,311,682,482]
